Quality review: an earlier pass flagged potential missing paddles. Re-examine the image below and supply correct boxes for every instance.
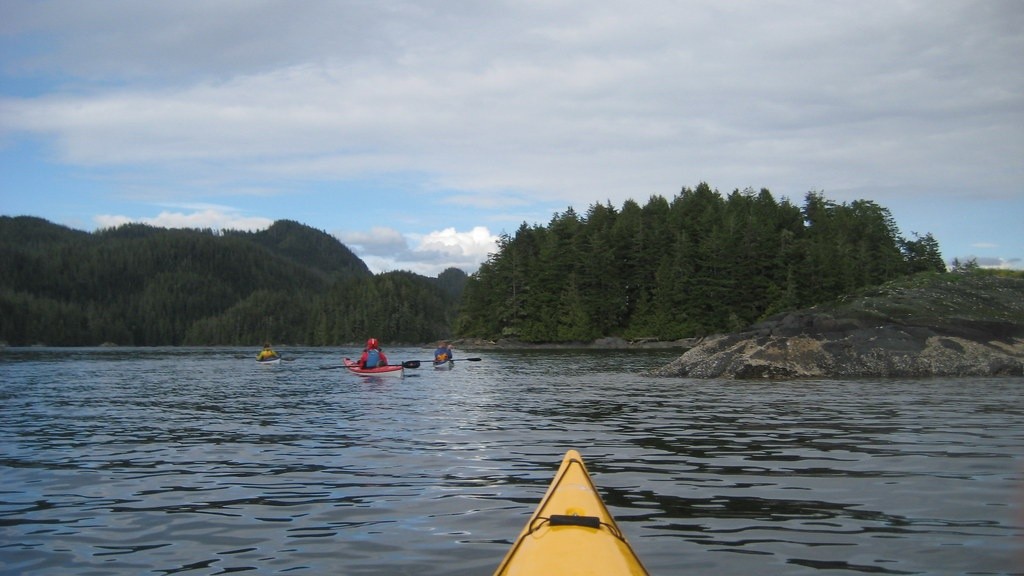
[407,358,481,363]
[234,355,294,361]
[319,361,420,369]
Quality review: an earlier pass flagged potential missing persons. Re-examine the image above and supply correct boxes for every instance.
[255,343,280,362]
[435,342,452,364]
[356,338,387,370]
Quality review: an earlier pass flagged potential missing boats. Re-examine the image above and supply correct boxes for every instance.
[343,357,404,378]
[254,355,282,364]
[433,359,454,368]
